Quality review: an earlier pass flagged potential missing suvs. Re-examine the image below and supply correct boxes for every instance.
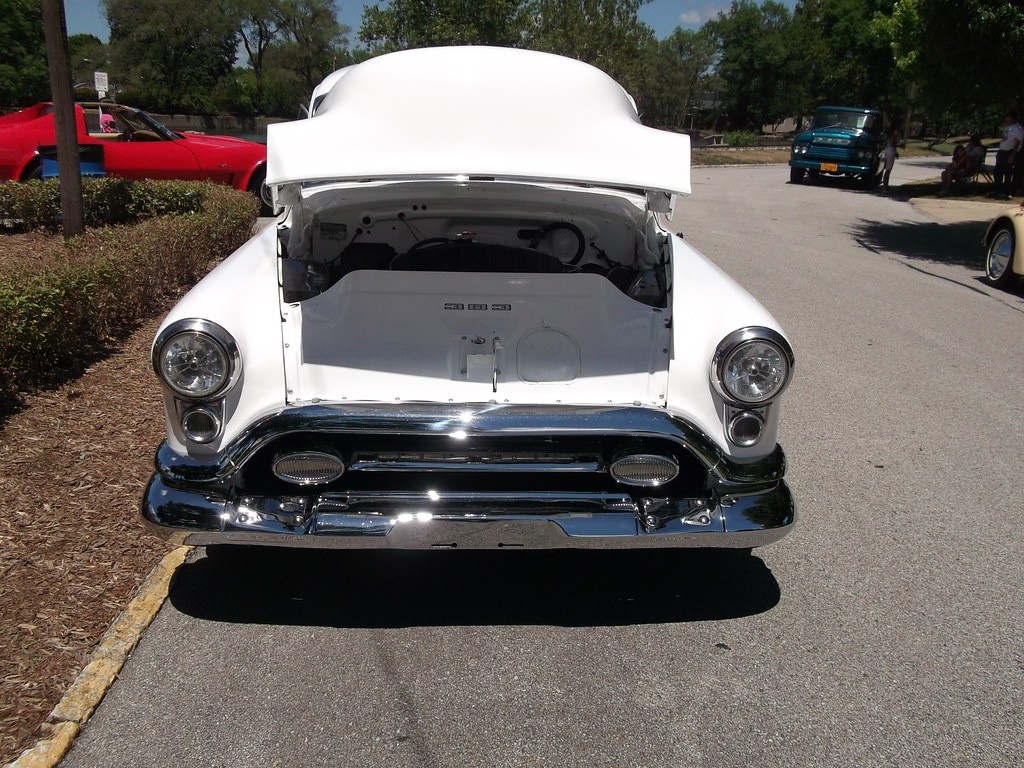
[787,104,899,191]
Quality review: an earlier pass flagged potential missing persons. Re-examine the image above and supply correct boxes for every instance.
[936,112,1024,200]
[100,115,117,134]
[882,119,897,188]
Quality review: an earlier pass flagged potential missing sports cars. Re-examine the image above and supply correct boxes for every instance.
[0,99,286,219]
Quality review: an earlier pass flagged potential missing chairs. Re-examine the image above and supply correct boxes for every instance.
[960,147,996,186]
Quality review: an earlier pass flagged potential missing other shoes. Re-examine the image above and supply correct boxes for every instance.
[1004,191,1012,200]
[986,190,1001,198]
[935,191,950,197]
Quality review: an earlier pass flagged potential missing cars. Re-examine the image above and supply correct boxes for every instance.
[127,44,798,573]
[980,199,1024,291]
